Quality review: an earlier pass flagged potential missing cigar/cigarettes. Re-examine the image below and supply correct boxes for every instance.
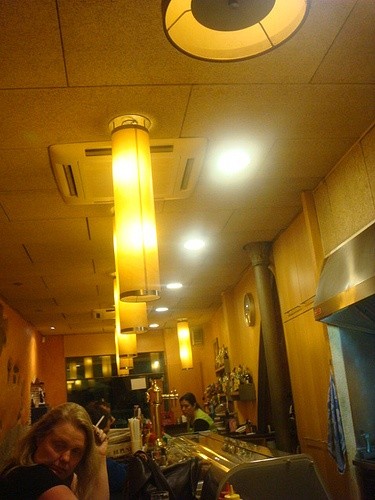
[96,416,104,428]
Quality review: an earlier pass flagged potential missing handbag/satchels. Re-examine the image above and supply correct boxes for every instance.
[128,449,202,500]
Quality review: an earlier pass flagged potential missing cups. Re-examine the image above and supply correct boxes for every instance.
[246,424,252,433]
[226,418,238,432]
[152,451,162,466]
[151,491,169,500]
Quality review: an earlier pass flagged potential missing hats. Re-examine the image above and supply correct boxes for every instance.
[86,403,110,429]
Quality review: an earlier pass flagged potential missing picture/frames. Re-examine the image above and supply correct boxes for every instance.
[212,337,219,357]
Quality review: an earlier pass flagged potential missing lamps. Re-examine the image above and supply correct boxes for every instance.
[177,316,194,370]
[111,273,148,334]
[161,0,310,63]
[108,113,161,304]
[115,304,137,376]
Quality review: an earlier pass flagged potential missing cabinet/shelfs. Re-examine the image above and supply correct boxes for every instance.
[214,354,256,416]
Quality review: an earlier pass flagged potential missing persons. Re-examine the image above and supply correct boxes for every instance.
[0,403,110,500]
[179,393,214,432]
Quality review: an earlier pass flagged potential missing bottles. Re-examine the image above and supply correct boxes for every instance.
[218,482,243,500]
[231,364,252,392]
[146,430,156,446]
[216,345,228,369]
[217,373,233,393]
[222,417,225,427]
